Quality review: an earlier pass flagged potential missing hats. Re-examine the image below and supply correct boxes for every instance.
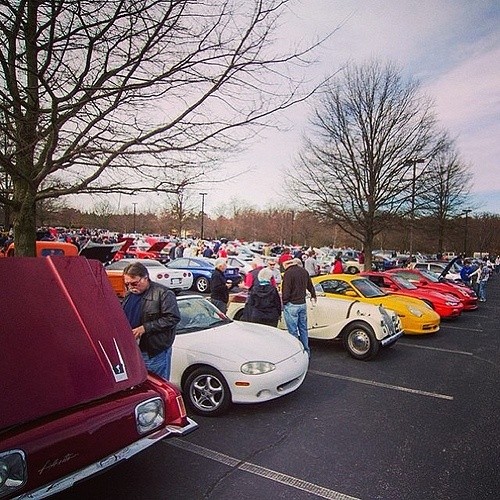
[280,255,294,264]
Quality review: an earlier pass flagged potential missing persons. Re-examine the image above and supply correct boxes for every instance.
[281,256,317,359]
[0,224,500,303]
[210,257,232,315]
[119,261,181,382]
[243,268,282,328]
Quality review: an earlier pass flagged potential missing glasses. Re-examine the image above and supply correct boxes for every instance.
[124,276,145,287]
[270,264,275,267]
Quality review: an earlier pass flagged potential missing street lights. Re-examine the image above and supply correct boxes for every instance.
[199,192,209,239]
[405,158,427,217]
[463,210,472,255]
[132,203,138,226]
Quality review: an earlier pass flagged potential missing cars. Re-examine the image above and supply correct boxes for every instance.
[0,227,500,361]
[168,295,309,416]
[0,255,199,500]
